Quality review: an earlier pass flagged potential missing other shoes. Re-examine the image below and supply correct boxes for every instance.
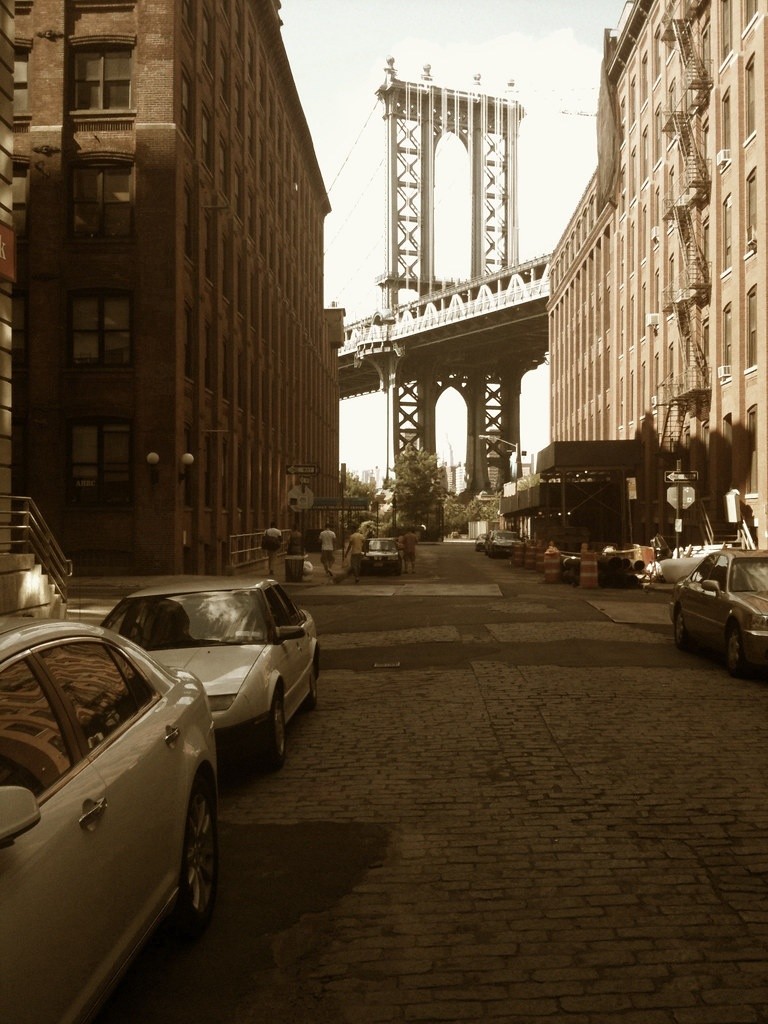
[355,576,360,584]
[270,570,273,575]
[412,572,415,574]
[328,568,333,576]
[404,572,409,574]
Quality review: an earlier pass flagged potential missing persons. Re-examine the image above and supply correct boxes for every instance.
[345,526,365,584]
[318,524,338,577]
[262,521,282,576]
[403,528,418,575]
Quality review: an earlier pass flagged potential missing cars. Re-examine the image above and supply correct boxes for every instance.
[361,538,402,576]
[96,578,320,770]
[475,534,486,551]
[0,618,218,1023]
[485,529,521,558]
[669,549,768,677]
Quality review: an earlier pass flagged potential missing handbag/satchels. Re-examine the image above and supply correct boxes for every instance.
[288,541,298,555]
[262,529,281,551]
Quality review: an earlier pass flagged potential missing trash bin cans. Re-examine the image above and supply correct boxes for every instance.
[284,556,304,581]
